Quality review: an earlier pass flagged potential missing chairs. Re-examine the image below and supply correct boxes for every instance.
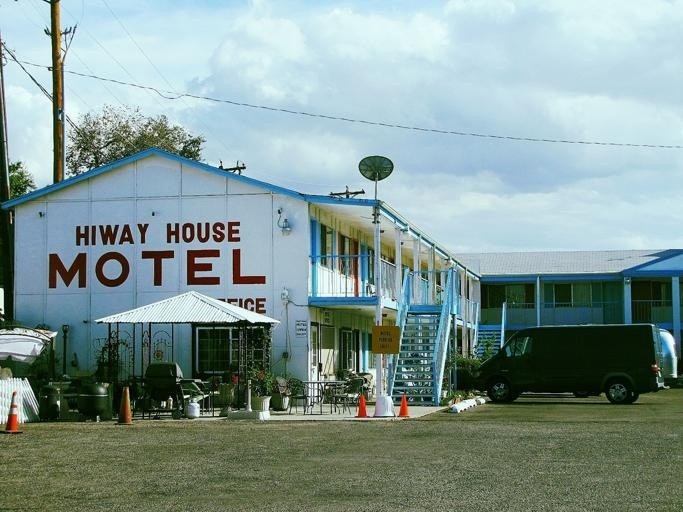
[275,371,373,415]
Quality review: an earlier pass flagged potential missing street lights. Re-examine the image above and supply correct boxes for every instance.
[356,156,393,403]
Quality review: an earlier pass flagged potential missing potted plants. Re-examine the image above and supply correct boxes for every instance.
[271,377,289,409]
[247,363,272,411]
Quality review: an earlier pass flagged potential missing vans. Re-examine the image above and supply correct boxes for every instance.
[471,321,666,405]
[656,327,678,382]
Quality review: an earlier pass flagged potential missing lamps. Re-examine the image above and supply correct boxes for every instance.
[282,219,290,232]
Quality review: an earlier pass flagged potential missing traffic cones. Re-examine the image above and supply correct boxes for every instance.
[0,391,23,435]
[397,394,410,418]
[354,395,370,419]
[115,385,137,426]
